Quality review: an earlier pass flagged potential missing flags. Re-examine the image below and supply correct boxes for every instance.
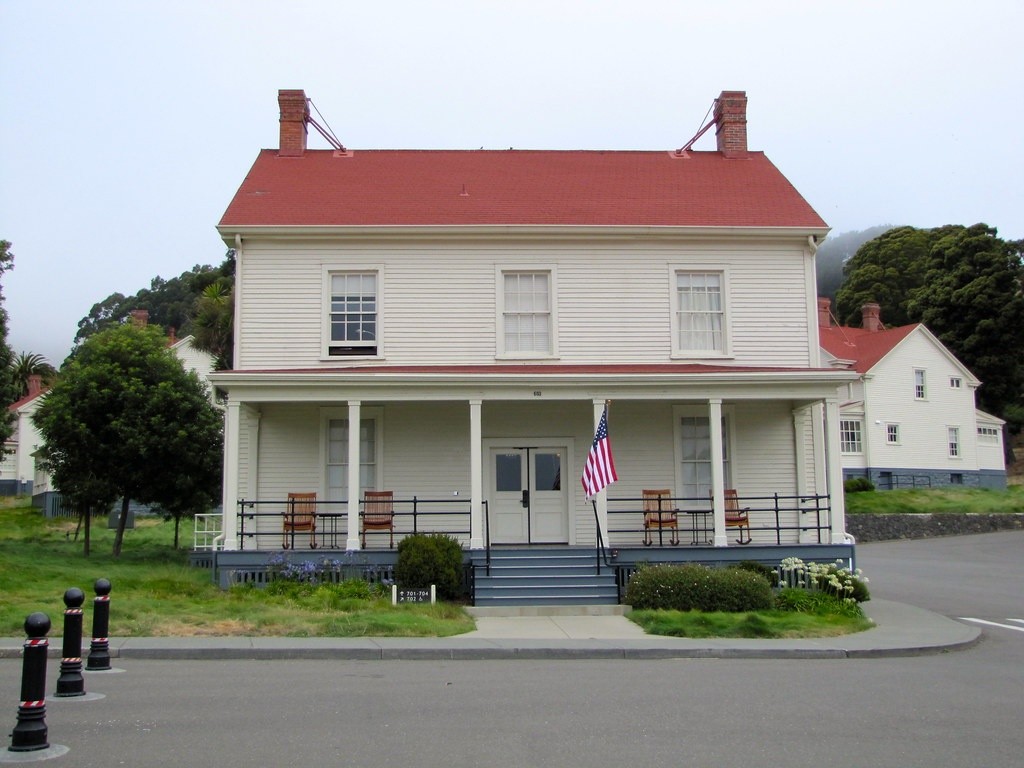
[581,406,618,505]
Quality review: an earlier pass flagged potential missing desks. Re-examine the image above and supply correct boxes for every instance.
[316,512,348,549]
[675,509,714,546]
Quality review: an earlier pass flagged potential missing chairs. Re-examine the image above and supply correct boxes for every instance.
[361,490,394,549]
[642,488,680,546]
[709,488,752,545]
[281,492,317,549]
